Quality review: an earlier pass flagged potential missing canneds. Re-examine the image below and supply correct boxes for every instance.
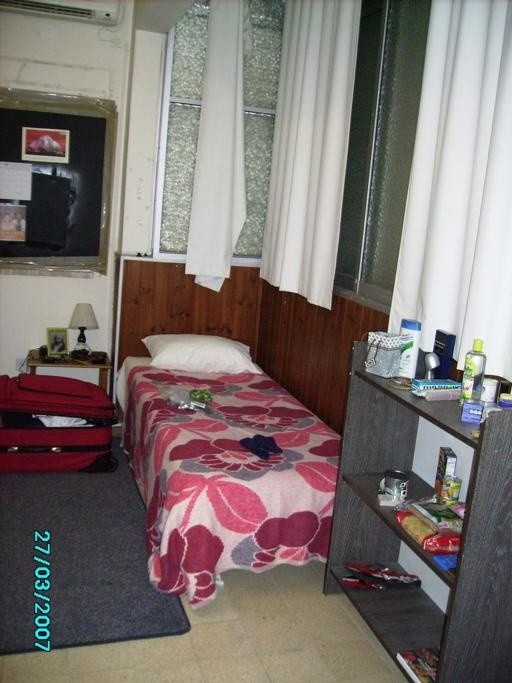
[385,470,409,499]
[441,476,460,509]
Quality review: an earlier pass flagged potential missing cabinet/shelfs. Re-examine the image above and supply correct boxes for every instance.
[322,340,512,683]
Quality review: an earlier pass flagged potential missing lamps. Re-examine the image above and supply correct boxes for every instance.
[68,303,99,353]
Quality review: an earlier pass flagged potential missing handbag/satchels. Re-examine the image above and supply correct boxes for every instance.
[365,332,401,379]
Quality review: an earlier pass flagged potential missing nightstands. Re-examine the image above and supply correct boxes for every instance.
[28,350,113,395]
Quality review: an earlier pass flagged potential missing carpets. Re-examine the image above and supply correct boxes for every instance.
[1,464,191,655]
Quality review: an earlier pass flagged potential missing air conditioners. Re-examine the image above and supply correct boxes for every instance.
[2,0,120,26]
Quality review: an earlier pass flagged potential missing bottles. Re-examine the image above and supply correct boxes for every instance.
[457,339,485,408]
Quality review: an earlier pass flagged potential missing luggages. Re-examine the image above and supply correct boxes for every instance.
[1,372,120,473]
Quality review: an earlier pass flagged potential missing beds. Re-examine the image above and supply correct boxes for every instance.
[118,356,342,610]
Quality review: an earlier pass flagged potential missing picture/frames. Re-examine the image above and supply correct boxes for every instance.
[47,327,68,358]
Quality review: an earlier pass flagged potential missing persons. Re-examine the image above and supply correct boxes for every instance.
[1,212,16,231]
[52,333,65,352]
[12,211,26,233]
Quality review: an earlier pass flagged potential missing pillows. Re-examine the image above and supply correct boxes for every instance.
[141,334,263,374]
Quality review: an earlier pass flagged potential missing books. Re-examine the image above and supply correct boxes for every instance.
[396,646,440,683]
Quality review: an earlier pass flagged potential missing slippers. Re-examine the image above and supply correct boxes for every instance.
[253,434,283,454]
[240,438,270,460]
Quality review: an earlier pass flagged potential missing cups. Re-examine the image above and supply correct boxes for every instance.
[377,470,410,500]
